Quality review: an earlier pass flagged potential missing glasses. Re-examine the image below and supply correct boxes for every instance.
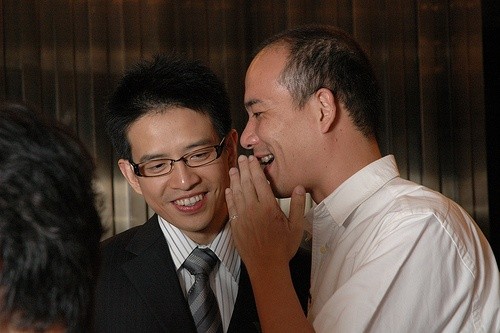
[128,133,227,178]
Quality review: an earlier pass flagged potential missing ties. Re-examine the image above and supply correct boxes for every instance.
[181,247,223,333]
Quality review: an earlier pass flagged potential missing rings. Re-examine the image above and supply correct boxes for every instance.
[229,215,239,223]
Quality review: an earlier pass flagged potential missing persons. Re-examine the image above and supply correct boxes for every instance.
[0,104,108,333]
[84,48,312,333]
[224,24,500,333]
